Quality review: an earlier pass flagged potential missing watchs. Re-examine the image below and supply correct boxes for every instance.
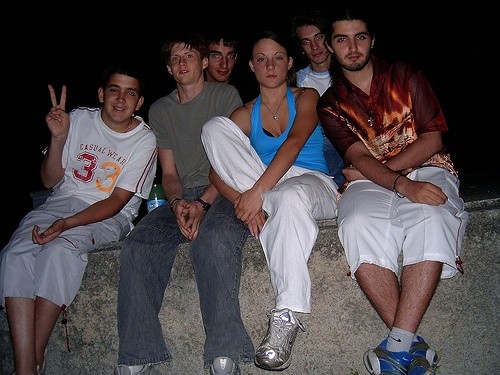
[194,197,211,213]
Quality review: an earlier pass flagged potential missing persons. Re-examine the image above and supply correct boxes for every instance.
[317,9,469,375]
[0,65,159,375]
[293,12,333,96]
[202,34,239,84]
[114,34,255,375]
[262,93,286,121]
[200,31,342,371]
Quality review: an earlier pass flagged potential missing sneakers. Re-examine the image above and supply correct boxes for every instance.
[253,308,306,370]
[363,336,411,375]
[407,336,441,375]
[113,362,155,375]
[209,357,241,375]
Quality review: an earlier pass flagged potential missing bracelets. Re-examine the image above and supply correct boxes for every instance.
[393,174,411,198]
[170,198,182,214]
